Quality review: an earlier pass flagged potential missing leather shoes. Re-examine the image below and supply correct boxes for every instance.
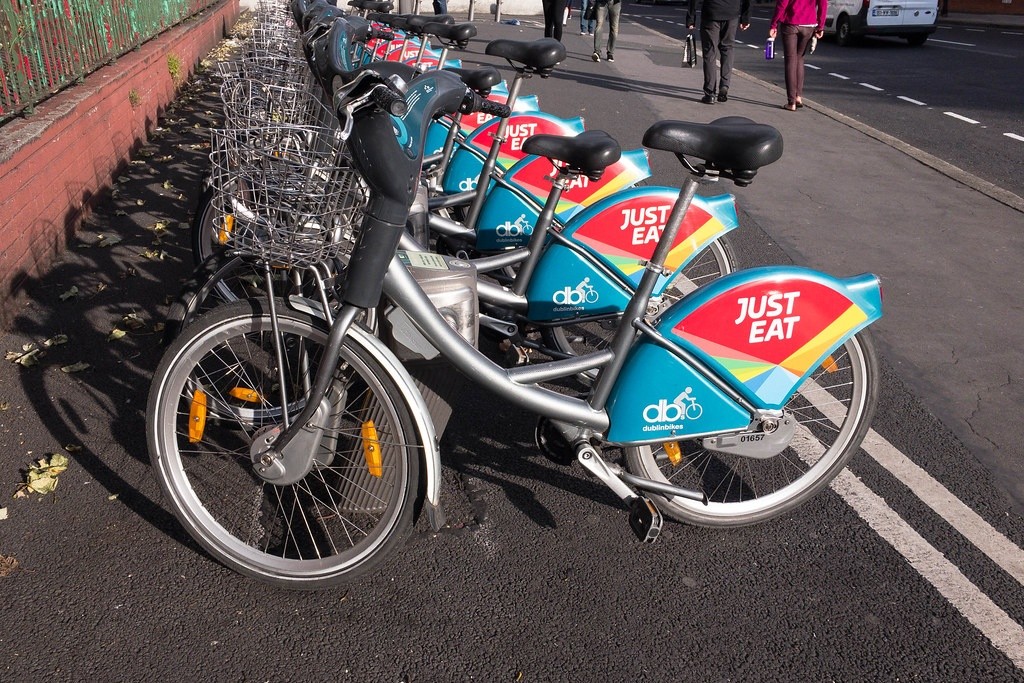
[717,94,727,102]
[702,95,715,104]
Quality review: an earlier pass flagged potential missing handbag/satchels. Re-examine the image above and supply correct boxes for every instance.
[583,0,596,20]
[809,35,817,55]
[681,30,697,69]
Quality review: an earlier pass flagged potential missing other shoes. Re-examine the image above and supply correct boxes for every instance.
[784,103,796,110]
[592,52,600,63]
[589,31,594,36]
[568,15,571,20]
[795,96,802,108]
[607,54,614,63]
[580,31,586,35]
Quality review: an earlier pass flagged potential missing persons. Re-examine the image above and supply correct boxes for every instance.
[542,0,572,64]
[686,0,752,104]
[579,0,621,62]
[768,0,828,111]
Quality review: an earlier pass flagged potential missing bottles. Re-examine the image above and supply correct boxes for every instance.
[765,38,775,59]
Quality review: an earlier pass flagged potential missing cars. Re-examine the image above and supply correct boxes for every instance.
[823,0,940,46]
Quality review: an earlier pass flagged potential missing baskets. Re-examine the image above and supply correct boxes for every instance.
[208,0,373,270]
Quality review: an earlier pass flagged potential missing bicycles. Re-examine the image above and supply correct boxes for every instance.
[147,71,885,589]
[161,0,738,428]
